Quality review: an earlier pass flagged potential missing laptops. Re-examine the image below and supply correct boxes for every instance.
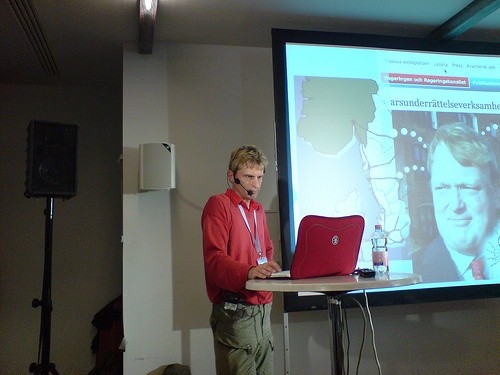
[266,215,366,280]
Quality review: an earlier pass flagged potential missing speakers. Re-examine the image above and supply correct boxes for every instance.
[139,142,175,191]
[24,120,79,199]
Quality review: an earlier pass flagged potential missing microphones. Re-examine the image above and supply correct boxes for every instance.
[240,183,253,195]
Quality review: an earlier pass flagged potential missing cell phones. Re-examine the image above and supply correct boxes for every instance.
[359,268,375,278]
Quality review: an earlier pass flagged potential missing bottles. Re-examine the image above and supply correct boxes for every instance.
[371,225,389,274]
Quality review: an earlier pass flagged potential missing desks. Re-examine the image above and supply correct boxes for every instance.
[245,271,423,375]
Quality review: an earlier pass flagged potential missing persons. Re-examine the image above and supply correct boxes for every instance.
[412,121,500,283]
[201,145,281,374]
